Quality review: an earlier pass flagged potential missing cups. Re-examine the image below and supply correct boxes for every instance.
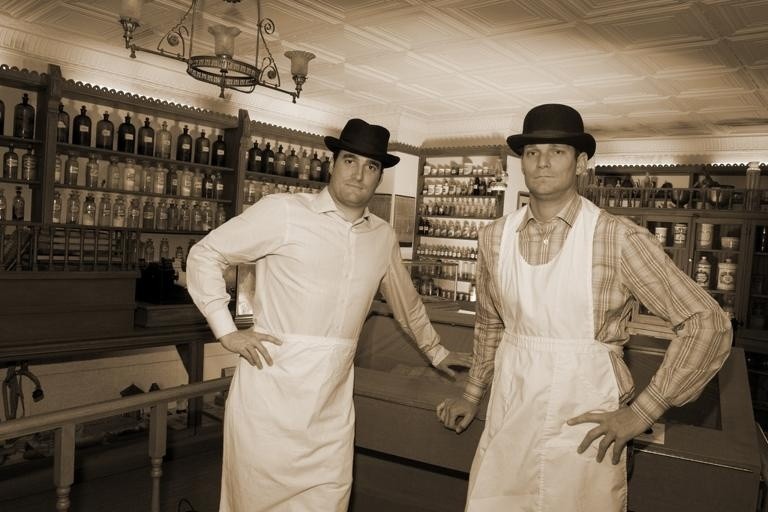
[695,224,714,249]
[655,227,670,246]
[673,224,688,248]
[720,236,739,250]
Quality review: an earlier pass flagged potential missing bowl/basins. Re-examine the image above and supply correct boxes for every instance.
[670,189,690,208]
[707,186,735,209]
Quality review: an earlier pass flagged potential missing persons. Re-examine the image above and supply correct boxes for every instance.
[437,104,734,512]
[185,118,474,512]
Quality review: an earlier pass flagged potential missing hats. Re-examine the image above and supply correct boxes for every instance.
[322,118,400,168]
[507,103,597,160]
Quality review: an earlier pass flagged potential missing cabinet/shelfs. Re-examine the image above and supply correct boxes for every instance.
[578,162,768,419]
[0,63,333,429]
[411,145,508,312]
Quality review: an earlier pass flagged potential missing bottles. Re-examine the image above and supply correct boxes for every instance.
[245,139,330,205]
[597,172,658,198]
[418,160,499,304]
[694,256,714,288]
[2,91,229,269]
[716,257,737,292]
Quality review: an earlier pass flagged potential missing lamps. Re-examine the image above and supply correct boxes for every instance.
[117,0,316,103]
